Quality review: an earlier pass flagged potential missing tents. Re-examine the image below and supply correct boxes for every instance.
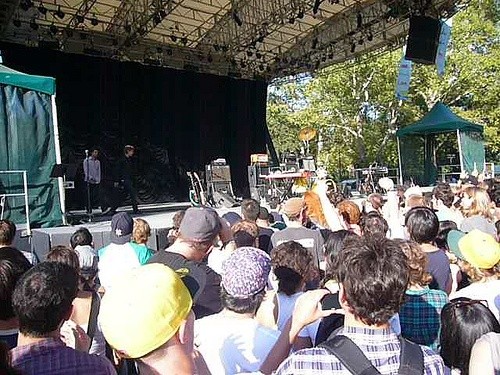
[0,64,66,226]
[396,100,484,185]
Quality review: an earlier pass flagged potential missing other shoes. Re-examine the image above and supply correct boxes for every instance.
[133,210,142,214]
[110,209,117,213]
[100,206,112,215]
[84,213,94,218]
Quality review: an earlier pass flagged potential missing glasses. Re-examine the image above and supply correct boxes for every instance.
[444,299,489,315]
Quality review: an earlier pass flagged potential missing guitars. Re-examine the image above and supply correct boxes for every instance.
[186,171,200,207]
[192,172,207,207]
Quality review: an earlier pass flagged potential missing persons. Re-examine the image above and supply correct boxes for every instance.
[83,148,111,215]
[0,168,500,375]
[110,145,144,215]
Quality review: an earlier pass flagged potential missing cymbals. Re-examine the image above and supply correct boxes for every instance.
[297,126,317,142]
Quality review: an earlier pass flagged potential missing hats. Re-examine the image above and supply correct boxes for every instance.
[447,229,500,269]
[284,198,307,217]
[222,247,271,297]
[125,145,135,149]
[180,207,232,242]
[110,212,134,244]
[74,245,98,273]
[403,185,423,198]
[100,263,192,357]
[218,212,243,245]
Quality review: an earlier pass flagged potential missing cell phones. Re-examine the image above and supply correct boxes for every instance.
[320,293,342,310]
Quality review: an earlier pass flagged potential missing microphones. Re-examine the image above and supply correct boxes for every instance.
[85,149,88,156]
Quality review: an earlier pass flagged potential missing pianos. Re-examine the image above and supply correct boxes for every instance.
[263,172,311,204]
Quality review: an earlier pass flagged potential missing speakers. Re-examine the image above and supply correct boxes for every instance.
[248,165,269,187]
[205,166,230,182]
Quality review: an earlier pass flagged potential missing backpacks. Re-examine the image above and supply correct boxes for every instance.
[315,285,345,345]
